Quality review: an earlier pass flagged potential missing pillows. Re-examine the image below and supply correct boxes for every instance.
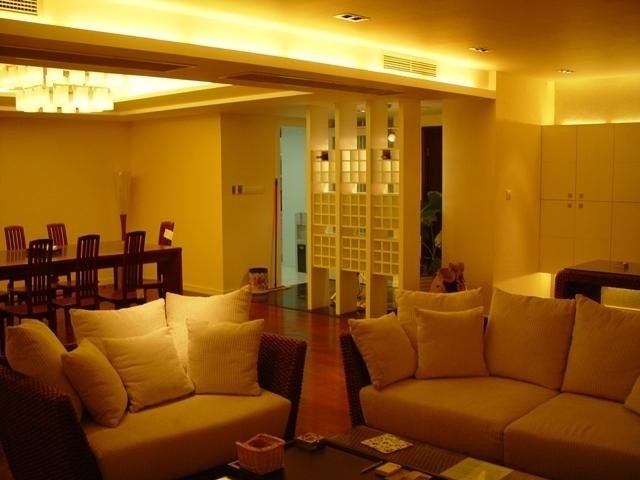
[187,319,265,395]
[165,284,252,374]
[104,326,195,413]
[484,288,576,390]
[392,288,487,354]
[69,298,168,359]
[561,295,640,401]
[61,337,128,428]
[411,304,490,379]
[622,377,640,416]
[5,319,82,426]
[348,313,418,390]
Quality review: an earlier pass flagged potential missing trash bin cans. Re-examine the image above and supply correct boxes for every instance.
[246,266,273,298]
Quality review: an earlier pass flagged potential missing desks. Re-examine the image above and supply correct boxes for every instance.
[554,259,640,304]
[0,242,182,327]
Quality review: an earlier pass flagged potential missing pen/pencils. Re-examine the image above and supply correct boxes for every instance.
[359,460,384,475]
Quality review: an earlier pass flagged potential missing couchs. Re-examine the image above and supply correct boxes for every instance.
[0,330,307,479]
[340,317,640,480]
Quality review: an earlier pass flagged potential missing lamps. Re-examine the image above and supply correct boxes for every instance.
[8,65,115,112]
[113,169,132,241]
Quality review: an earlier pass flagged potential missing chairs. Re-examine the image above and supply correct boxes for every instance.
[4,225,57,305]
[47,224,101,296]
[98,230,146,311]
[143,221,175,302]
[53,235,100,335]
[0,239,55,356]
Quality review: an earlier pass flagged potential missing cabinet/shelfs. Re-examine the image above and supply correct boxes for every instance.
[538,124,614,282]
[611,122,640,263]
[310,145,401,280]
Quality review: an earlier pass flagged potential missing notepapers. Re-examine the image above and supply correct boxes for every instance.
[376,462,404,474]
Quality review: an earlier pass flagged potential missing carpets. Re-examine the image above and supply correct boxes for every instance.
[329,426,544,480]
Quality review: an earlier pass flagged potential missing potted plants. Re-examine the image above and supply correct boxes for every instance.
[420,190,442,274]
